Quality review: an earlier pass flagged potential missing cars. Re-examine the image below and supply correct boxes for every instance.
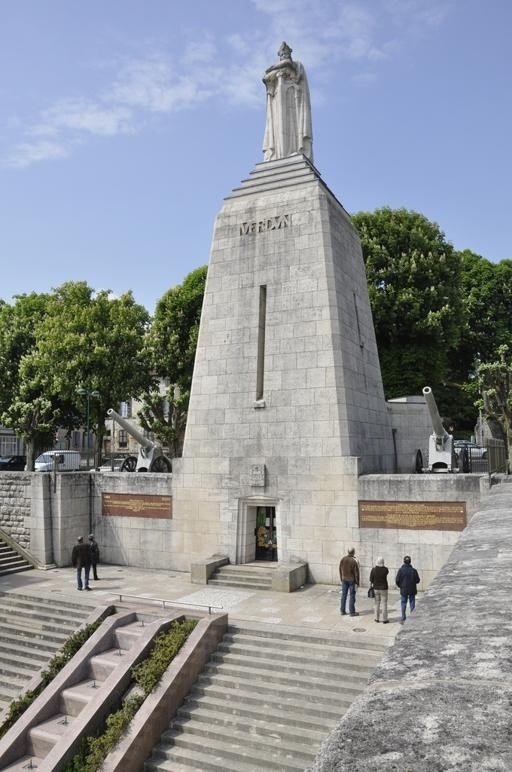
[452,439,488,460]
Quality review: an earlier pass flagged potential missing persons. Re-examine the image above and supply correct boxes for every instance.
[262,41,313,163]
[369,557,389,623]
[339,546,359,616]
[71,536,92,590]
[396,556,420,625]
[86,534,100,580]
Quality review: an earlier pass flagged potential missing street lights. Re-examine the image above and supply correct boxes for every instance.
[467,373,483,443]
[78,388,99,471]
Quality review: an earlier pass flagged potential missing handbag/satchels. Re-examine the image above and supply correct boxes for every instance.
[368,587,374,598]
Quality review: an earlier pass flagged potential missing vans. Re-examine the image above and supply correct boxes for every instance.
[0,455,35,471]
[34,450,81,472]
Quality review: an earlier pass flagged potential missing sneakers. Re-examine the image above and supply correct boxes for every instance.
[374,619,389,623]
[94,577,99,579]
[341,611,358,616]
[78,587,91,590]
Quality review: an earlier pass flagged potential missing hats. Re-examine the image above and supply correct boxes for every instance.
[376,557,384,567]
